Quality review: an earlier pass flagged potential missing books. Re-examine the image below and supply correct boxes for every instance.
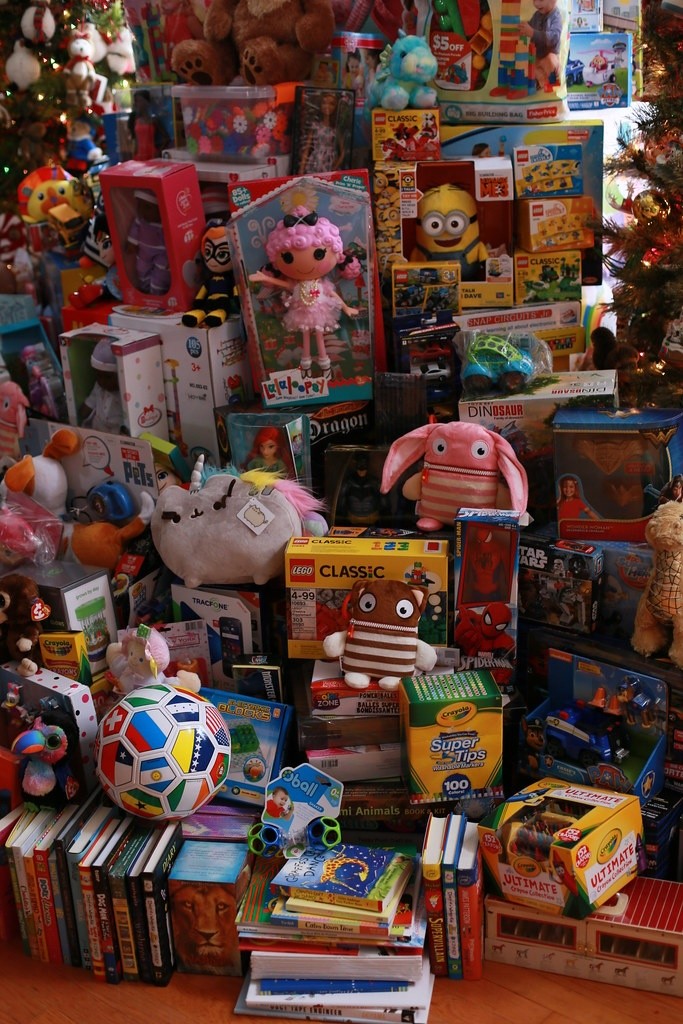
[0,783,185,987]
[234,811,487,1024]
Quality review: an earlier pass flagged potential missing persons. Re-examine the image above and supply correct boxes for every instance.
[249,206,359,380]
[556,476,600,523]
[342,48,364,97]
[299,94,345,174]
[240,426,297,482]
[77,335,124,434]
[127,90,171,161]
[266,786,294,820]
[125,189,171,296]
[465,528,510,602]
[518,0,562,91]
[337,455,388,526]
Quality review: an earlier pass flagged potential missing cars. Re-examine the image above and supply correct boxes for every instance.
[543,704,634,771]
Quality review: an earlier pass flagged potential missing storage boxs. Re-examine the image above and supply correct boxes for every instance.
[0,0,683,999]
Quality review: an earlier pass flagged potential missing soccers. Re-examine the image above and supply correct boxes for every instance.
[92,682,231,822]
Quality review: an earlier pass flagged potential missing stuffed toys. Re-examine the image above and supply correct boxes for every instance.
[366,28,438,112]
[380,422,528,532]
[171,0,337,86]
[151,454,329,589]
[10,708,87,813]
[323,579,437,691]
[182,222,241,328]
[5,0,136,309]
[106,624,201,696]
[631,500,683,671]
[0,380,154,677]
